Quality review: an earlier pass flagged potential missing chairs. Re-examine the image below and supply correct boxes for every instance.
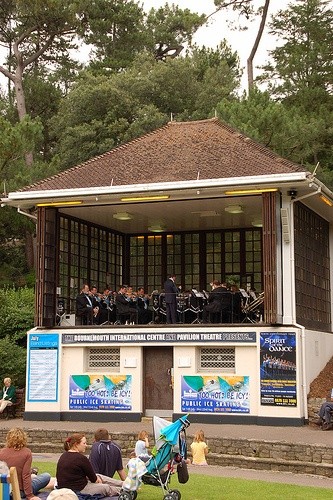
[77,305,134,325]
[210,298,231,322]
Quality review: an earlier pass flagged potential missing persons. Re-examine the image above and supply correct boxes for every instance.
[135,430,152,458]
[88,428,125,481]
[0,427,56,500]
[0,378,17,413]
[54,434,121,497]
[201,280,264,324]
[263,354,296,380]
[173,457,191,464]
[76,284,165,325]
[315,388,333,431]
[47,488,79,500]
[164,274,182,324]
[190,430,209,466]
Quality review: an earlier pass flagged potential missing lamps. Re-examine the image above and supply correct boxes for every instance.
[320,194,333,207]
[226,206,245,213]
[113,212,134,220]
[250,219,262,228]
[37,200,83,207]
[224,188,278,193]
[148,225,166,232]
[121,195,169,202]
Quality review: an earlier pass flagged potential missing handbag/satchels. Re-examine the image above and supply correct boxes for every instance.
[176,461,189,484]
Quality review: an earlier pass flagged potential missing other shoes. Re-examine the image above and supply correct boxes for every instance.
[30,466,39,475]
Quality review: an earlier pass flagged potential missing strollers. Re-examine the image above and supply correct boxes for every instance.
[119,414,190,500]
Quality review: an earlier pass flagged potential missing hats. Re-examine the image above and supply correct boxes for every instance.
[46,488,79,500]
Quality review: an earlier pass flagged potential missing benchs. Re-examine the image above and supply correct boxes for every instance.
[0,389,25,420]
